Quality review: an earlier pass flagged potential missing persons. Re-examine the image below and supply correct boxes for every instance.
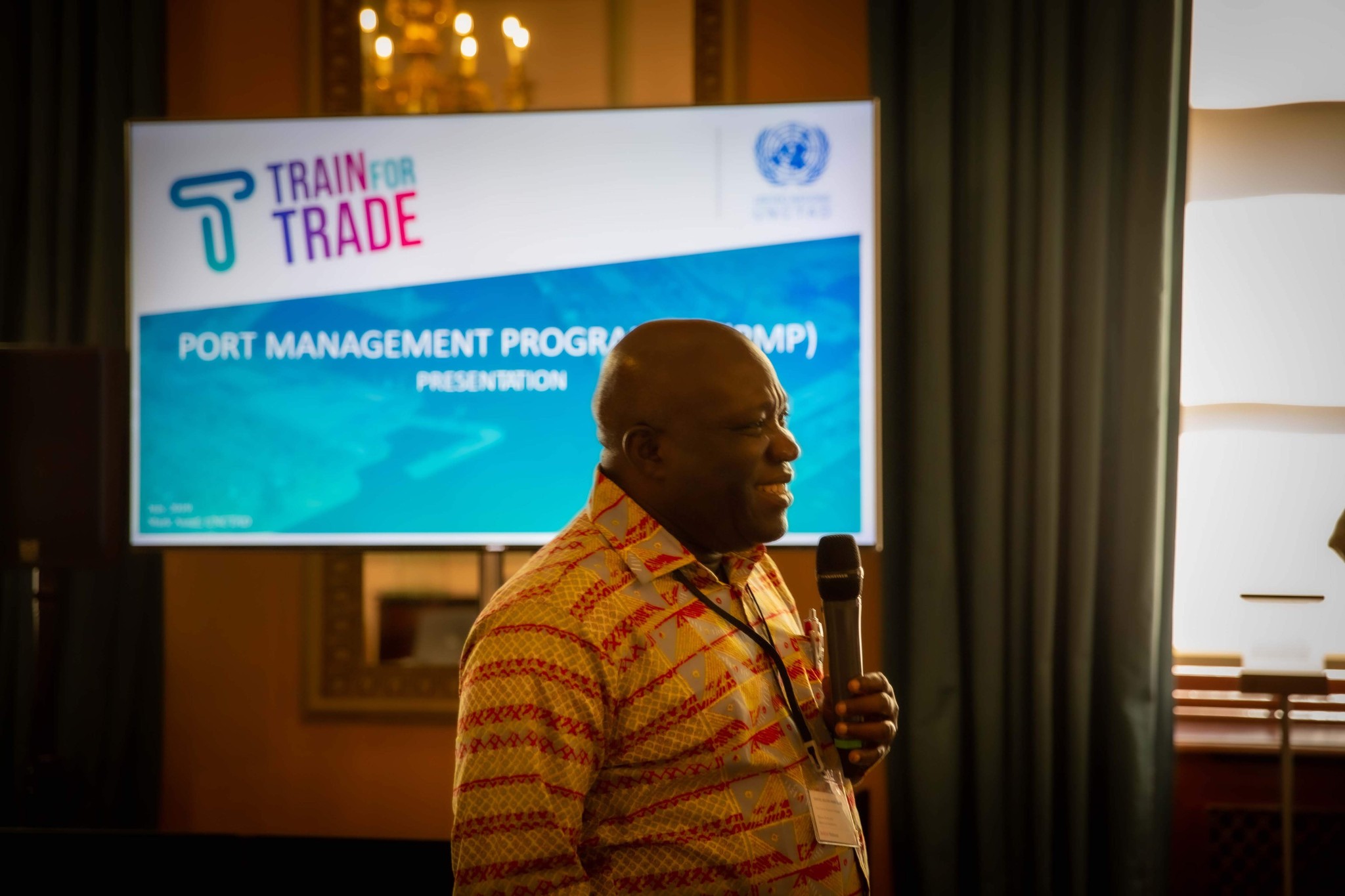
[451,320,900,896]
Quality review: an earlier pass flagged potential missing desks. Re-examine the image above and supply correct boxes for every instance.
[1170,720,1345,896]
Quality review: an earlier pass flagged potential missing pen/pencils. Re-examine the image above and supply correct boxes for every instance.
[808,608,824,660]
[803,619,818,667]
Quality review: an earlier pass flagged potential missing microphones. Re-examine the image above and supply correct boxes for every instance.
[815,534,865,776]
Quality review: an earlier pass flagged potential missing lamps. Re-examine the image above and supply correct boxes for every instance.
[355,0,547,117]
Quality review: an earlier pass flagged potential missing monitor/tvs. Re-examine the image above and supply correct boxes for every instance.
[123,95,886,557]
